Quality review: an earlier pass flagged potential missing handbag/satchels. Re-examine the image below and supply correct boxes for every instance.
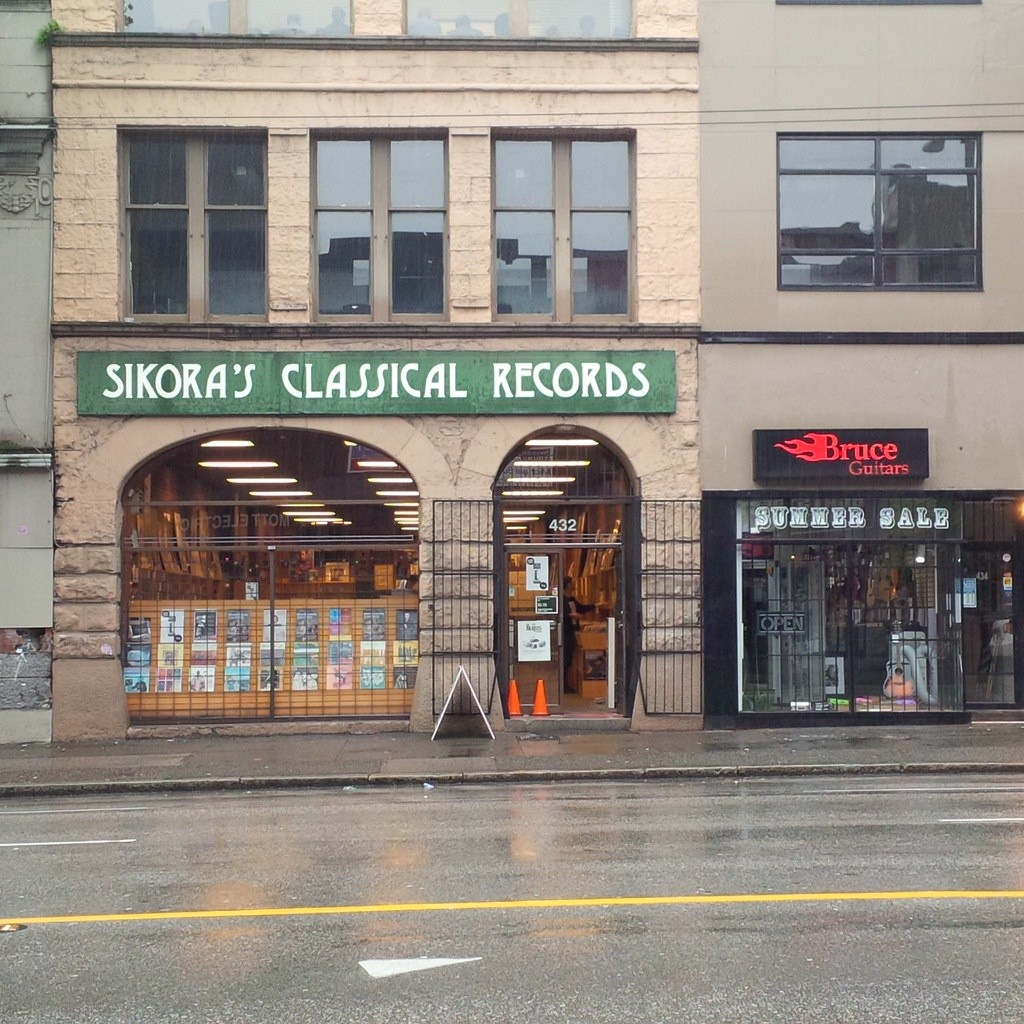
[887,674,914,697]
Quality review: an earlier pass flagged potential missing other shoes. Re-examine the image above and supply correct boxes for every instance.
[564,687,575,693]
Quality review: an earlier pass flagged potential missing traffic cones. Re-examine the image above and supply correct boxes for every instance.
[507,679,524,717]
[529,679,551,716]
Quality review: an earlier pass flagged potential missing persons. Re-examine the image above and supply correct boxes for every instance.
[132,612,418,689]
[293,550,314,581]
[552,577,607,693]
